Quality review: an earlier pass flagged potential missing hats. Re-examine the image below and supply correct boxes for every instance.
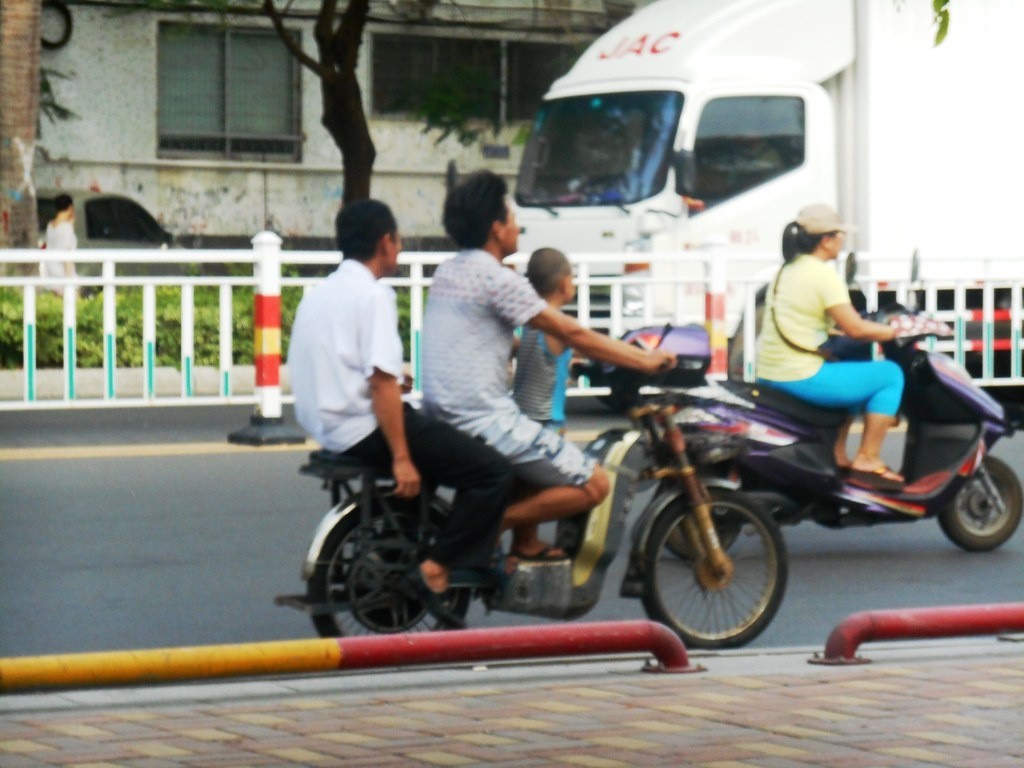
[795,203,858,237]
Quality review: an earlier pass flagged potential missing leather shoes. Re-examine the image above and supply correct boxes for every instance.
[396,566,466,630]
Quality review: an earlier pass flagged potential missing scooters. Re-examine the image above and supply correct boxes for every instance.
[592,250,1024,552]
[271,322,788,650]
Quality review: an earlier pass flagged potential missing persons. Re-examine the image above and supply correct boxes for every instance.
[730,113,781,172]
[514,247,577,559]
[757,205,908,490]
[40,193,80,299]
[422,170,675,584]
[286,198,512,632]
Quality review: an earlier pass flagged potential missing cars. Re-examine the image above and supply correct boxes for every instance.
[36,186,205,278]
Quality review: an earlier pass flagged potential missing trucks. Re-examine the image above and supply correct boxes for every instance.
[506,1,1023,416]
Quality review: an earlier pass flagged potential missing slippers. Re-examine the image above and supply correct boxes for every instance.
[848,467,904,488]
[510,545,569,561]
